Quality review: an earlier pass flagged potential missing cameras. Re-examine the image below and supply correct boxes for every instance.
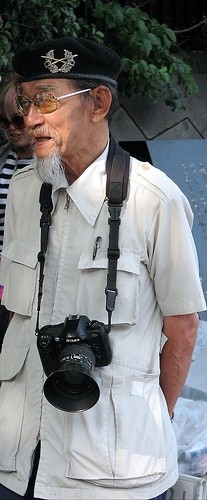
[37,313,112,413]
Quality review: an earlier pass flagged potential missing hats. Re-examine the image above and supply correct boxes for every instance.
[12,37,122,86]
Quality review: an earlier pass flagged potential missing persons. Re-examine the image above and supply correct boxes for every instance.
[0,73,34,256]
[0,36,207,500]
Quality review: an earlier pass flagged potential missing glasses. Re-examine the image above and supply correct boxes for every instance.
[0,113,26,129]
[15,88,91,116]
